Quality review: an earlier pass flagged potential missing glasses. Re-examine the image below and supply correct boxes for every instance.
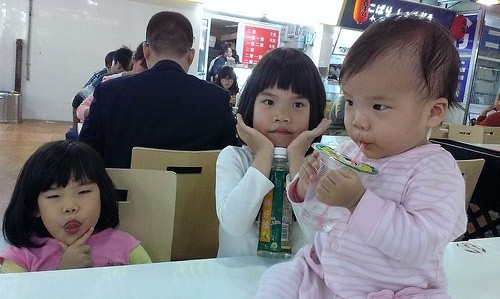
[221,78,234,82]
[105,65,111,69]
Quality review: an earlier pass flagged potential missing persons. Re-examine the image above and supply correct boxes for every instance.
[1,139,153,274]
[215,15,467,298]
[72,11,241,168]
[476,93,500,127]
[328,62,340,82]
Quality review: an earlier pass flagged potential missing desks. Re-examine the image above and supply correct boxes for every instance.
[0,236,500,299]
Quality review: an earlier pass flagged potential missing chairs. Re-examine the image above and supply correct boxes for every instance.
[429,124,500,211]
[105,146,223,262]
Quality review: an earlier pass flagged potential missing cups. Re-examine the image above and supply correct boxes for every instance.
[301,142,380,232]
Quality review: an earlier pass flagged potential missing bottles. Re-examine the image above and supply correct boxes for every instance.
[256,146,295,259]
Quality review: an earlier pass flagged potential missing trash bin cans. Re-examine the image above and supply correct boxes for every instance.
[0,90,23,124]
